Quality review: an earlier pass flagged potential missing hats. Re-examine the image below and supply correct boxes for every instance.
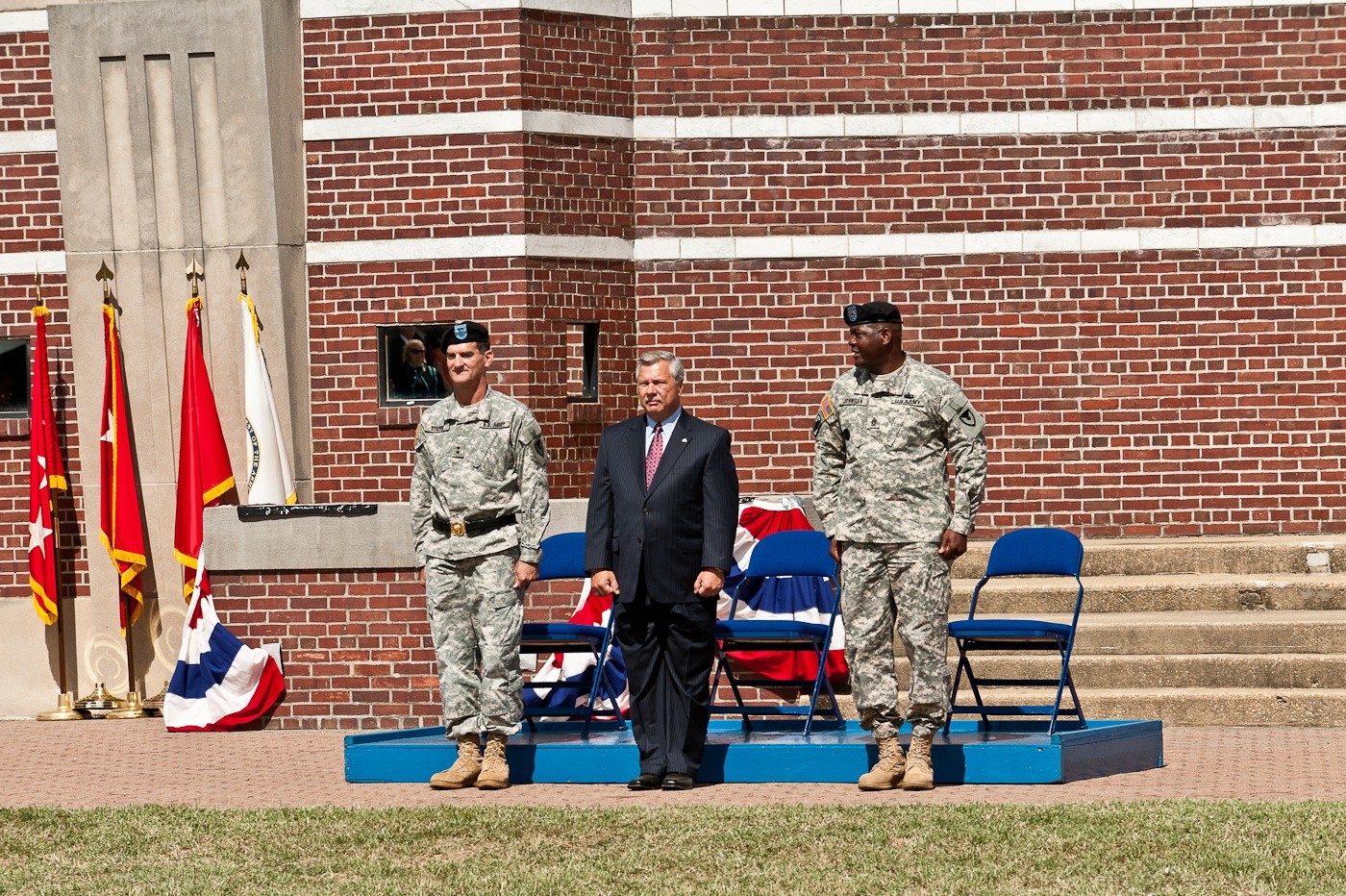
[441,321,489,354]
[843,301,903,327]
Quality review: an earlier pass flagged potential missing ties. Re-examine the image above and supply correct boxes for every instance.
[646,422,663,492]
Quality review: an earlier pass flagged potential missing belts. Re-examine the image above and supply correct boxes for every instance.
[432,513,516,538]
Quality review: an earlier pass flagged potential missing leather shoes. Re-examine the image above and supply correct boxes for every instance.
[627,772,662,789]
[660,773,693,790]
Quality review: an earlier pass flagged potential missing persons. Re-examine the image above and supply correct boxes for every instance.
[387,325,455,399]
[811,303,986,791]
[410,321,550,792]
[586,350,740,790]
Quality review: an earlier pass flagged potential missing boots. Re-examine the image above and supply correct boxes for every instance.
[476,733,510,790]
[858,735,907,789]
[901,735,934,790]
[430,732,483,790]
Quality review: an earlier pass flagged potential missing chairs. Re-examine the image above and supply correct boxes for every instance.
[943,528,1089,736]
[705,530,845,736]
[520,531,628,739]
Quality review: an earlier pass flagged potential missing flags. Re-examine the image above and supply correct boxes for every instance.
[236,293,299,509]
[171,298,236,607]
[97,304,149,637]
[25,306,67,625]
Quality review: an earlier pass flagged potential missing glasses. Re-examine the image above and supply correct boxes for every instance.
[408,346,424,354]
